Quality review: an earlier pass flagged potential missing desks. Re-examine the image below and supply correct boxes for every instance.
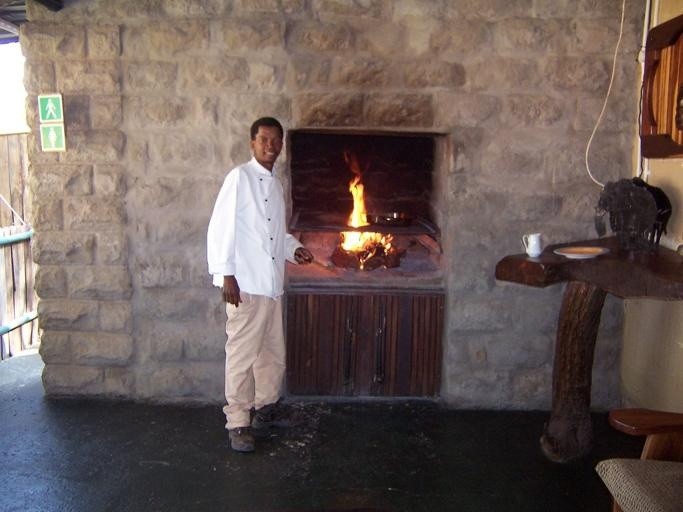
[495,233,683,465]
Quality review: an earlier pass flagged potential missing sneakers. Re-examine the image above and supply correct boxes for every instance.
[228,428,255,452]
[252,406,304,430]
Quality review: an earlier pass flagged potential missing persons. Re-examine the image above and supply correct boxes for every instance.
[205,117,315,453]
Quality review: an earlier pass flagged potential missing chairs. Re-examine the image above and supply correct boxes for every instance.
[594,409,683,512]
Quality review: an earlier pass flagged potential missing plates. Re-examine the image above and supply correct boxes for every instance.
[552,246,610,261]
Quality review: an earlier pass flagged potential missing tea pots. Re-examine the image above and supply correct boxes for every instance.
[521,232,550,259]
